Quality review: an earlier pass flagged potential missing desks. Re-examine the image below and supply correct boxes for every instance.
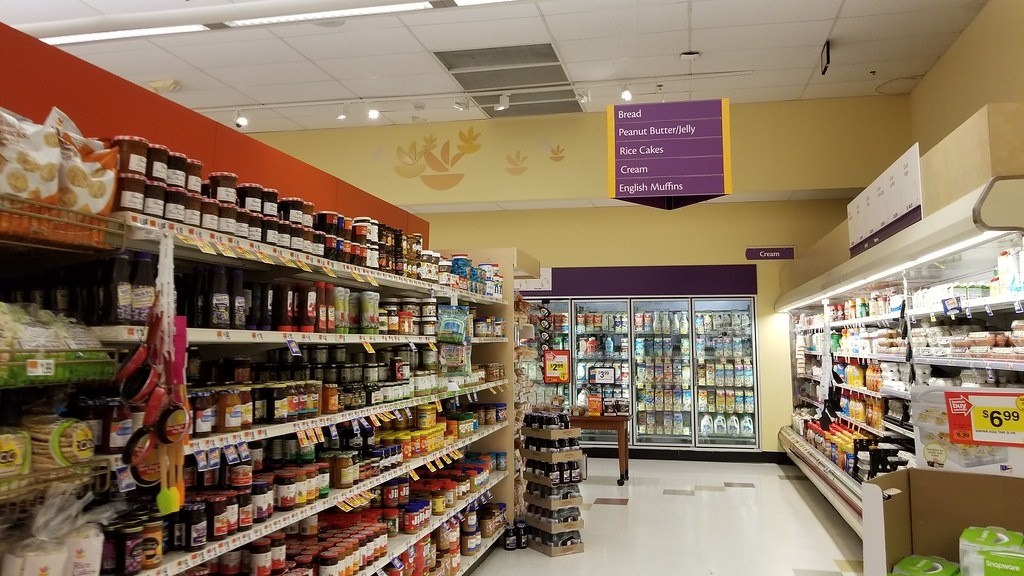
[568,415,633,487]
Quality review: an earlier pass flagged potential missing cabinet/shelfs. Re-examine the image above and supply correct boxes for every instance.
[0,23,756,576]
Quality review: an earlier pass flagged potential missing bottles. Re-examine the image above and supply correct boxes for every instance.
[652,312,688,333]
[578,384,590,406]
[800,328,823,351]
[700,413,754,435]
[588,337,613,354]
[506,411,582,550]
[838,328,877,353]
[834,358,883,392]
[805,422,881,476]
[793,313,823,325]
[799,380,819,403]
[840,389,887,431]
[845,291,890,319]
[6,248,380,335]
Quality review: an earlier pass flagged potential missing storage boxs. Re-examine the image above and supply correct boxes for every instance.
[521,426,581,440]
[528,538,584,557]
[522,471,583,487]
[525,516,584,534]
[522,449,582,464]
[959,525,1024,576]
[892,554,960,576]
[524,493,582,511]
